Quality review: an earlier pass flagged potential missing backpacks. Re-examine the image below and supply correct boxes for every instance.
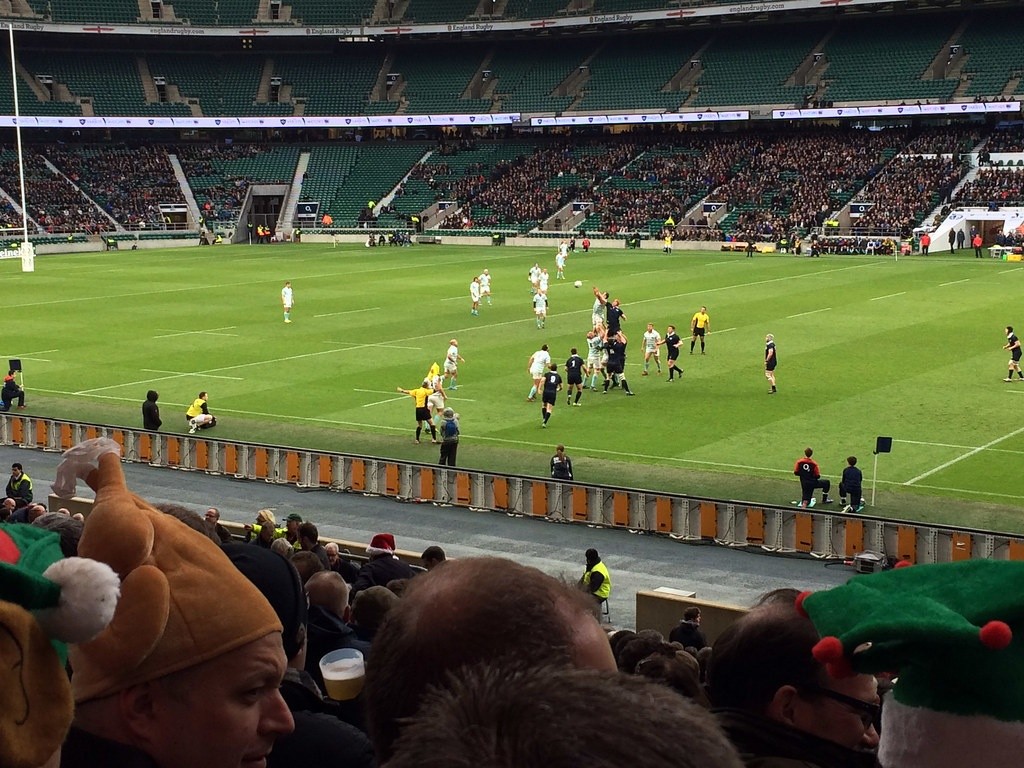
[445,419,456,435]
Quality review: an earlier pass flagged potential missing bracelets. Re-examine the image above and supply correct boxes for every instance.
[765,360,768,362]
[401,389,404,393]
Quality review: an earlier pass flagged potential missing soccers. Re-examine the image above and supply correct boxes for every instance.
[574,280,583,289]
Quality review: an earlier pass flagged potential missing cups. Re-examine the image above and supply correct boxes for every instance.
[318,648,367,701]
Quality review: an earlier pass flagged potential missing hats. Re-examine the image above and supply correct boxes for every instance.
[797,561,1024,768]
[365,533,399,561]
[283,513,302,521]
[47,439,286,708]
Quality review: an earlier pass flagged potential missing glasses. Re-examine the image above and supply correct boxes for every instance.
[801,682,881,730]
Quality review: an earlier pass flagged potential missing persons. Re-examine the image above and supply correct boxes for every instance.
[690,306,711,354]
[528,263,549,330]
[565,348,589,406]
[656,325,684,382]
[527,344,552,402]
[538,364,563,428]
[0,123,1024,259]
[581,288,627,396]
[397,339,466,466]
[281,282,294,324]
[1003,326,1024,382]
[641,323,661,375]
[469,269,493,315]
[0,437,1024,768]
[2,370,28,408]
[764,334,778,393]
[143,390,162,430]
[554,251,567,280]
[186,392,212,431]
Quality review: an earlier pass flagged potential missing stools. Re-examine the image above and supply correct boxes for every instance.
[602,599,612,623]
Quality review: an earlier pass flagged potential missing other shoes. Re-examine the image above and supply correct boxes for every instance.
[582,385,589,389]
[666,378,675,382]
[573,402,581,406]
[822,499,834,504]
[541,418,547,428]
[526,394,538,402]
[590,386,598,392]
[839,501,846,506]
[769,387,777,394]
[642,371,648,376]
[602,390,608,394]
[626,391,635,396]
[1017,377,1024,381]
[656,370,662,375]
[1003,377,1012,382]
[567,396,571,405]
[679,368,684,378]
